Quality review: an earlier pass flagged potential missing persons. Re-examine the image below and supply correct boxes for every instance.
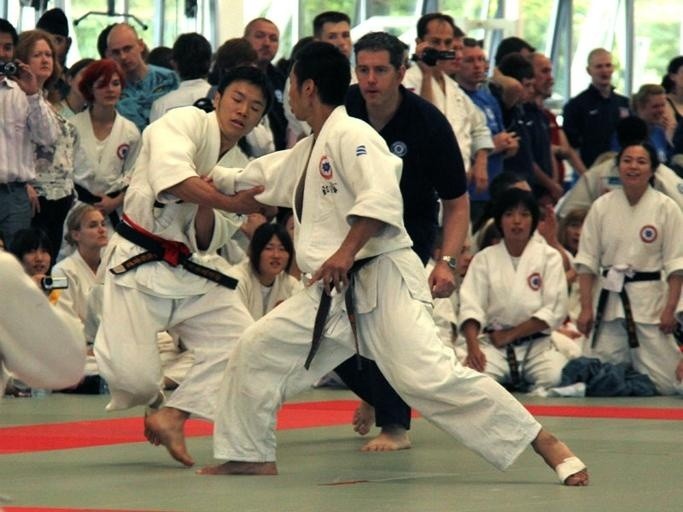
[335,32,470,452]
[93,64,274,468]
[194,42,590,487]
[0,8,683,394]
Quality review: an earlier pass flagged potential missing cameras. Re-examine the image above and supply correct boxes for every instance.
[0,56,19,77]
[422,47,455,67]
[39,276,68,294]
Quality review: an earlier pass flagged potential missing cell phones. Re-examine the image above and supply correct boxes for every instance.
[507,120,524,131]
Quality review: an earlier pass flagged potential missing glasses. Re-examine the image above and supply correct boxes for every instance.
[463,38,484,47]
